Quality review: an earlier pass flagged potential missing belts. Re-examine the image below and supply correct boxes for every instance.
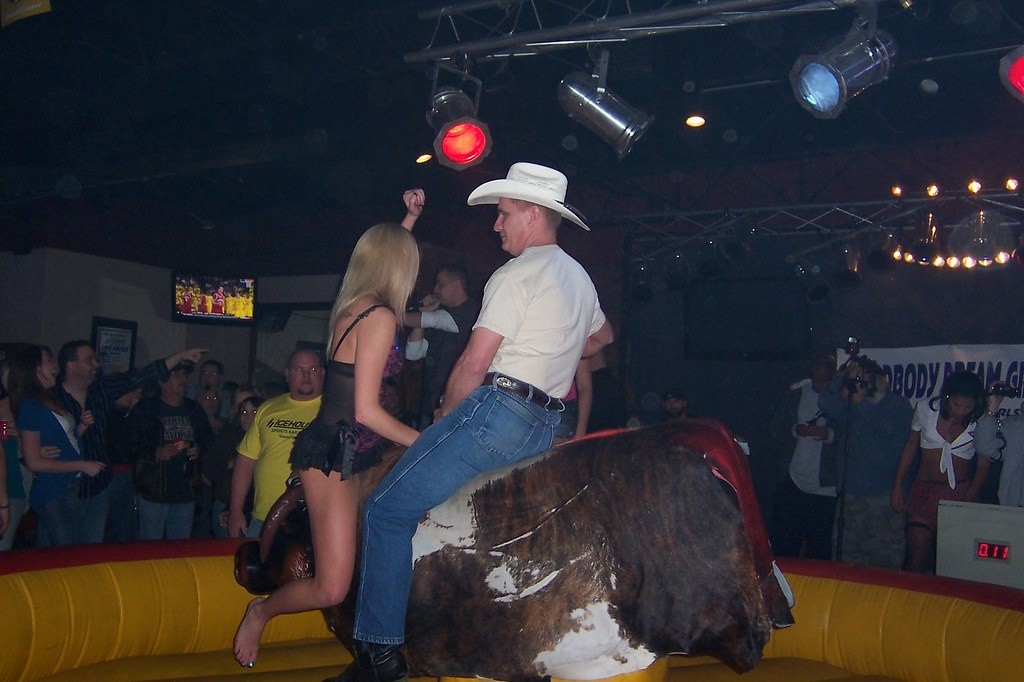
[481,372,558,411]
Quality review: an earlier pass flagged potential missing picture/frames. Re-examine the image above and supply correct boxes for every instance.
[90,315,138,379]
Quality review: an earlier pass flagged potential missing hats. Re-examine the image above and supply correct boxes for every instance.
[466,161,591,231]
[662,387,688,401]
[152,358,194,374]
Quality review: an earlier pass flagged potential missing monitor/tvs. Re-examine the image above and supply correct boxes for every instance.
[171,270,258,326]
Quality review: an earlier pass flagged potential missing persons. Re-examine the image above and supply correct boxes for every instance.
[233,188,425,668]
[376,377,419,450]
[553,346,1024,582]
[398,264,480,431]
[0,341,283,550]
[320,162,613,682]
[227,345,325,538]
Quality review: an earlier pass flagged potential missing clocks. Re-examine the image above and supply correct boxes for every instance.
[632,260,662,306]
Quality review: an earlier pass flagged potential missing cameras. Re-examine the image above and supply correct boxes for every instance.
[994,384,1016,398]
[840,338,867,393]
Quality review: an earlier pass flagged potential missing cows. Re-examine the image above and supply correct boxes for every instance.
[232,419,773,682]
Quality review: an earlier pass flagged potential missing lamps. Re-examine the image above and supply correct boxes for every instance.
[422,54,494,173]
[556,46,654,159]
[790,4,899,119]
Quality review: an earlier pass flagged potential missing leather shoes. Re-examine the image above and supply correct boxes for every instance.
[321,641,408,682]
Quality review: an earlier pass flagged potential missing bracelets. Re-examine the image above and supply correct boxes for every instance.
[986,409,996,418]
[0,505,10,509]
